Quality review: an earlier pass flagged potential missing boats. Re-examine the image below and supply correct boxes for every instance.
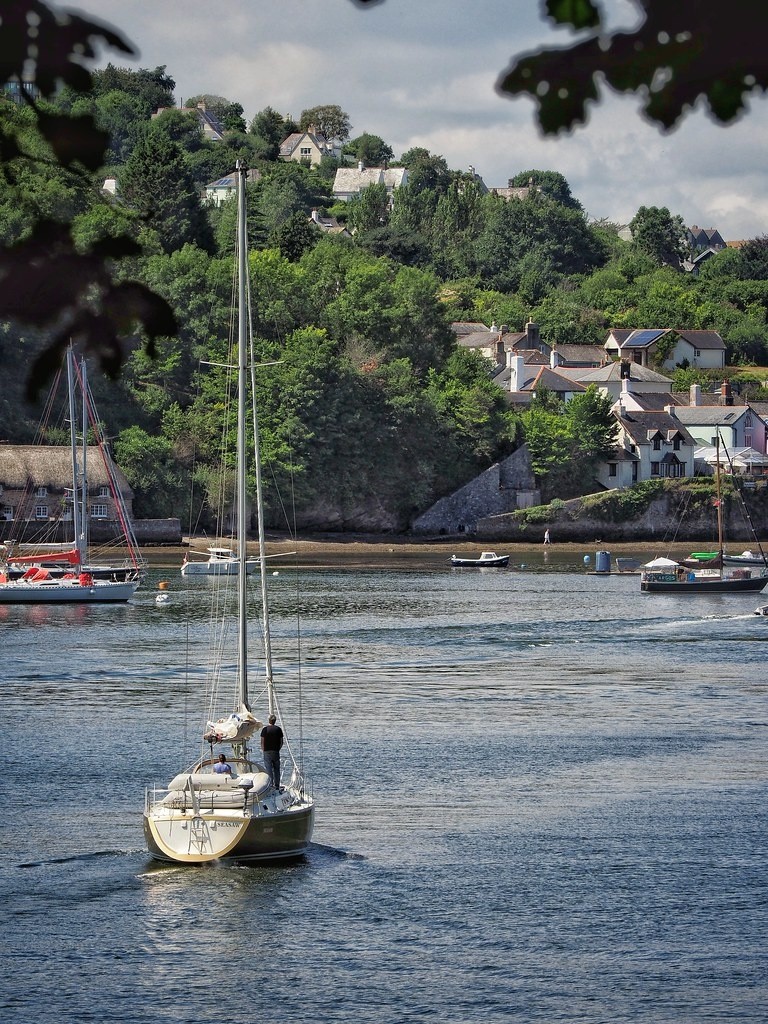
[691,551,719,562]
[179,549,299,576]
[444,551,510,568]
[721,550,768,568]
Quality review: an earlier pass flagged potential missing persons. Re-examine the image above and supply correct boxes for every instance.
[543,529,551,545]
[260,715,284,792]
[213,753,232,774]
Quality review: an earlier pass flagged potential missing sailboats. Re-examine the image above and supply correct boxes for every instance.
[637,424,768,596]
[141,160,323,870]
[0,340,150,606]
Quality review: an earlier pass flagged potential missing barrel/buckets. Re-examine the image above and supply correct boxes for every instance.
[596,551,611,571]
[687,573,695,581]
